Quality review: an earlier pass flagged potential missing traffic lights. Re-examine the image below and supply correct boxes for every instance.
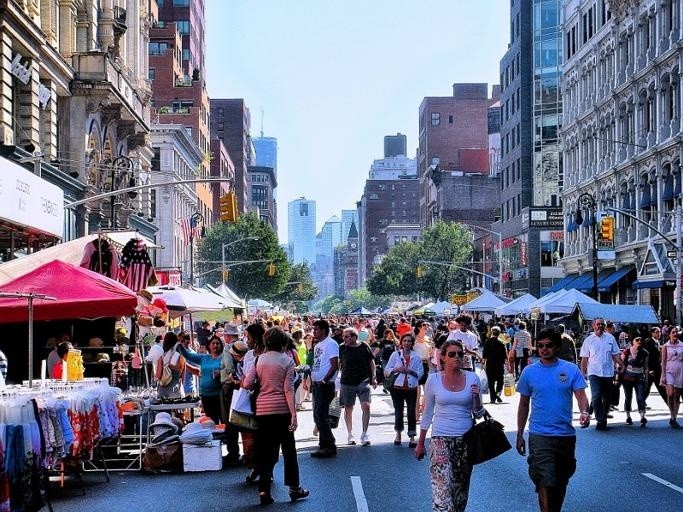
[268,264,274,276]
[415,266,421,277]
[219,194,229,221]
[597,215,614,241]
[298,285,303,292]
[222,270,228,281]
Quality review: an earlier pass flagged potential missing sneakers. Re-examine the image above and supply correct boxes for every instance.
[348,435,356,445]
[608,406,680,427]
[394,439,401,445]
[223,449,338,483]
[409,443,417,448]
[361,435,370,446]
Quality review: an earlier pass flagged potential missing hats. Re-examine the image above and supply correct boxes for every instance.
[46,337,57,349]
[147,412,226,447]
[137,289,168,327]
[229,341,247,356]
[144,335,157,344]
[89,338,103,345]
[221,323,241,335]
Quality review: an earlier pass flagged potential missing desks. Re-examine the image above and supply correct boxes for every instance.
[82,400,152,474]
[153,395,200,429]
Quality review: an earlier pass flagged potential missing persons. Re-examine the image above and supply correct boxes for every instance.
[1,312,683,512]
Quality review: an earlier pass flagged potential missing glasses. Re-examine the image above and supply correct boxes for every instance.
[536,342,554,348]
[636,339,643,342]
[446,352,464,358]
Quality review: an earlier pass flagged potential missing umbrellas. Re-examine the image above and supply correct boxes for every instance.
[1,260,272,322]
[345,288,601,315]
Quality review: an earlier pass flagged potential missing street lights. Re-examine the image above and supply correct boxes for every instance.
[189,211,207,287]
[459,221,503,297]
[109,153,137,229]
[573,192,596,301]
[220,235,260,283]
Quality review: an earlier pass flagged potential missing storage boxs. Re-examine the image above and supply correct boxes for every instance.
[184,439,223,473]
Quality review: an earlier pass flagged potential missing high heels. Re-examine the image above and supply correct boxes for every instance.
[259,490,274,506]
[289,487,309,500]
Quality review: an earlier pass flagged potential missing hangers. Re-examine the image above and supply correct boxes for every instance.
[0,382,125,412]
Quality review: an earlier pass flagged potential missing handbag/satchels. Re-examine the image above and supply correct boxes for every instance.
[159,366,173,387]
[228,383,259,433]
[516,346,524,358]
[504,377,515,396]
[383,373,398,390]
[463,419,512,465]
[293,371,309,404]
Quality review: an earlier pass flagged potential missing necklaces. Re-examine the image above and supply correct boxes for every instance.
[445,370,461,390]
